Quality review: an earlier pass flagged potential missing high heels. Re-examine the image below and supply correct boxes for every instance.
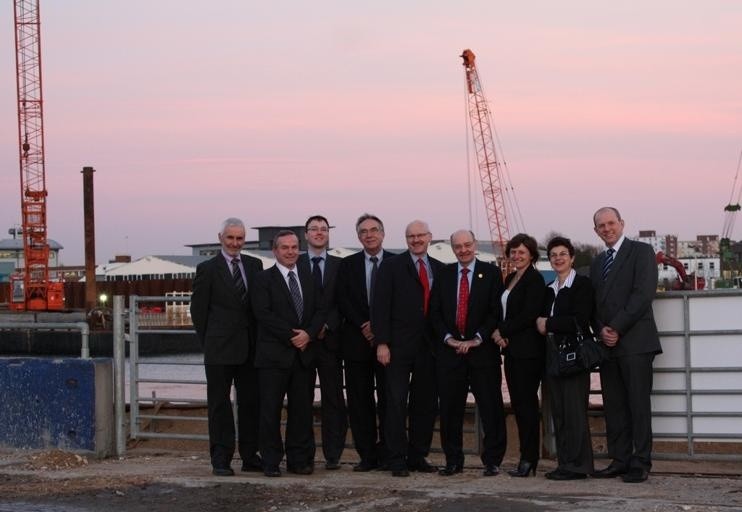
[506,460,538,477]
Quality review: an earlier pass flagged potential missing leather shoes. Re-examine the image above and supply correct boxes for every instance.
[623,465,646,482]
[214,463,234,476]
[241,459,264,472]
[439,464,463,475]
[591,461,626,478]
[483,464,498,476]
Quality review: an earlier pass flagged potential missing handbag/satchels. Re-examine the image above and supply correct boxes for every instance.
[545,324,600,379]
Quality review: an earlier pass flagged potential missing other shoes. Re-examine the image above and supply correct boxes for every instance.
[264,465,281,476]
[287,464,310,474]
[325,461,339,469]
[545,468,585,480]
[378,461,391,470]
[391,464,409,476]
[353,461,375,471]
[408,460,437,473]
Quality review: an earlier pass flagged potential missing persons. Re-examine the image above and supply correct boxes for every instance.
[371,219,448,477]
[425,227,506,478]
[294,214,347,473]
[246,228,319,476]
[333,213,400,474]
[189,218,262,478]
[585,207,666,485]
[534,235,596,481]
[492,233,547,480]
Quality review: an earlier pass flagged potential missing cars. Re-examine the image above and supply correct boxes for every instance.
[82,305,130,331]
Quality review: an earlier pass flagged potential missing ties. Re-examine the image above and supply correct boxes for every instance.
[370,256,379,302]
[313,259,323,299]
[288,271,303,322]
[604,250,614,285]
[232,257,248,302]
[457,269,470,336]
[417,258,431,313]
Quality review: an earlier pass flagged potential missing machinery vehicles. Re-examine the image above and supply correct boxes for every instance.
[7,0,64,312]
[654,250,707,292]
